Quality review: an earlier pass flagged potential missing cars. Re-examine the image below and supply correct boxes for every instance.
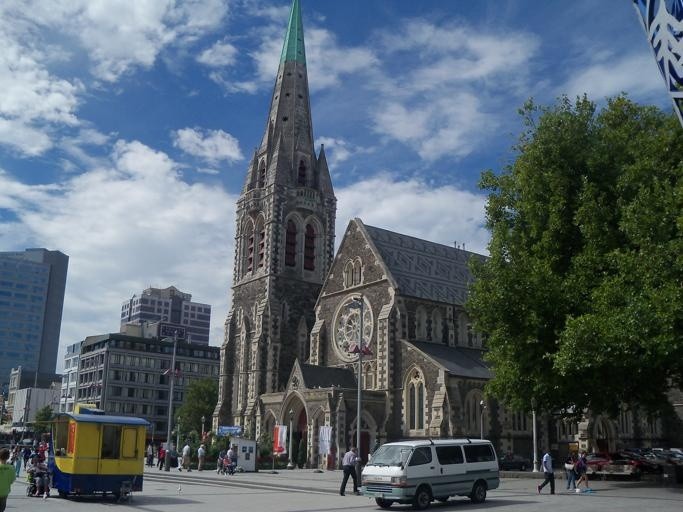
[499,454,531,471]
[576,447,683,471]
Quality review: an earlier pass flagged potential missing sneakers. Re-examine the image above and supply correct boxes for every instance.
[537,486,541,494]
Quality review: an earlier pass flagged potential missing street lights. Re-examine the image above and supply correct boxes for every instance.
[159,329,180,472]
[176,415,182,453]
[287,408,295,469]
[200,416,207,437]
[478,400,489,440]
[529,397,542,473]
[343,294,373,487]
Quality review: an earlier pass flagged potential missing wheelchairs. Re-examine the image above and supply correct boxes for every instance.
[221,461,236,476]
[24,469,52,498]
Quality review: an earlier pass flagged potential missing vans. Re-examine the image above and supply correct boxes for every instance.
[360,433,501,511]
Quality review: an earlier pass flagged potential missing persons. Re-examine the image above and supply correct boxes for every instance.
[339,447,360,496]
[565,451,580,493]
[147,443,237,476]
[0,439,53,512]
[537,448,555,495]
[576,451,592,491]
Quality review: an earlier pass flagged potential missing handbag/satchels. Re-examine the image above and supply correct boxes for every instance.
[564,457,574,471]
